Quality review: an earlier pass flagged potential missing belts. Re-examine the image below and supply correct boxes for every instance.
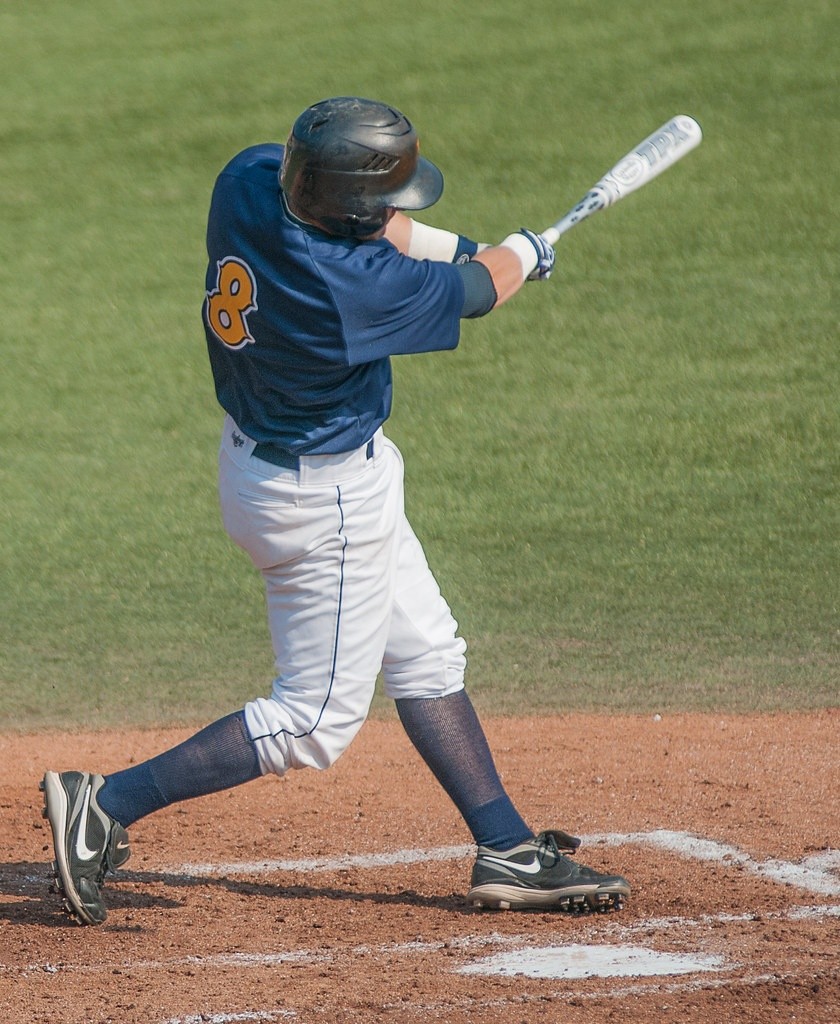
[251,438,374,471]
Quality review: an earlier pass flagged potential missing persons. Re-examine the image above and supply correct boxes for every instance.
[37,96,630,925]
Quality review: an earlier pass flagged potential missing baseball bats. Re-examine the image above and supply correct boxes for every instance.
[541,114,702,245]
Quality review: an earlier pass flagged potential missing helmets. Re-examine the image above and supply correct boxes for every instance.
[278,96,445,239]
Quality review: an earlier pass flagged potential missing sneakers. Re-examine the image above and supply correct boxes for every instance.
[464,830,631,916]
[37,770,130,925]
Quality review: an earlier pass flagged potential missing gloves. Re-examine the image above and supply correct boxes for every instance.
[506,227,557,281]
[450,231,495,265]
